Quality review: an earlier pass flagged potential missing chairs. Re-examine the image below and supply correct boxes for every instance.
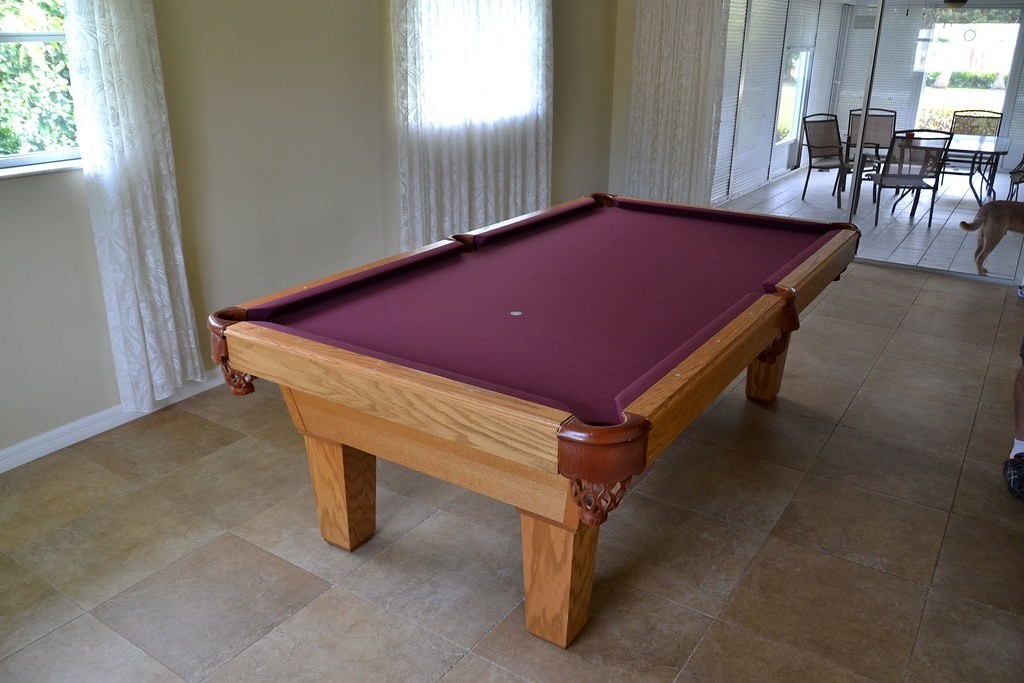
[852,128,955,227]
[941,110,1004,189]
[802,112,858,209]
[843,107,898,202]
[1006,153,1024,201]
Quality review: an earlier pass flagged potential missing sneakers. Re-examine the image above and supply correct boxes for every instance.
[1002,445,1024,499]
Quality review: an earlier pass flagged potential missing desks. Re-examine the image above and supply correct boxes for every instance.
[207,192,861,647]
[894,133,1011,218]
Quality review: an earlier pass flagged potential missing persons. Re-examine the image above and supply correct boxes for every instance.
[1004,336,1024,499]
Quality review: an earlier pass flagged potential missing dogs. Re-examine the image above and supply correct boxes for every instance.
[960,201,1024,277]
